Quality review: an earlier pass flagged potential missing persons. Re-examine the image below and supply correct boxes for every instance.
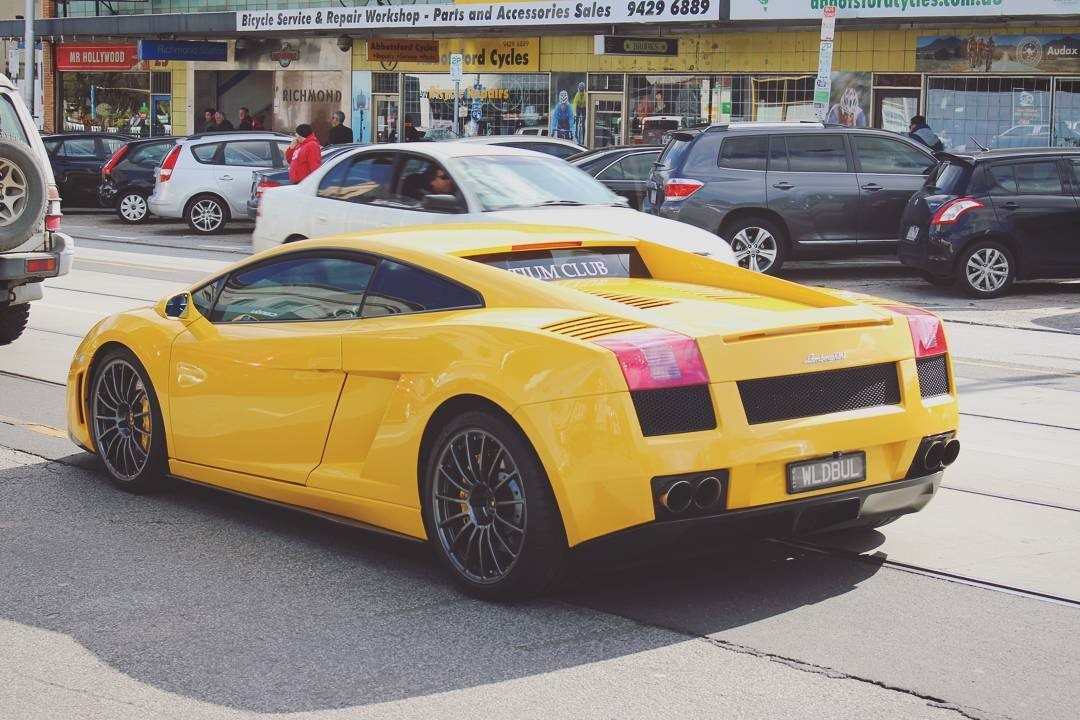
[827,88,866,127]
[240,108,264,130]
[372,159,392,183]
[552,82,587,145]
[967,34,994,71]
[327,111,353,144]
[285,124,322,184]
[406,117,419,141]
[418,164,455,203]
[909,116,943,150]
[205,108,233,132]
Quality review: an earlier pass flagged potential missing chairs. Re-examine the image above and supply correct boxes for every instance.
[405,172,424,200]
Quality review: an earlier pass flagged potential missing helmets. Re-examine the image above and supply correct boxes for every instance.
[579,82,586,90]
[559,90,568,103]
[841,88,858,114]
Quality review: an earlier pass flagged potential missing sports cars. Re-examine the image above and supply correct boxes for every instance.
[64,223,961,604]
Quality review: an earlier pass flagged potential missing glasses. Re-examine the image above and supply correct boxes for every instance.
[433,174,449,180]
[843,112,854,116]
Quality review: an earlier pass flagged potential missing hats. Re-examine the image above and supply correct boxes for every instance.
[296,124,313,138]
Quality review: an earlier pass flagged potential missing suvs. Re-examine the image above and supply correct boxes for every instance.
[642,119,942,279]
[999,124,1053,139]
[0,74,75,345]
[34,131,325,235]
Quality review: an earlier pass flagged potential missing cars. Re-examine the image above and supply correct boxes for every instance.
[898,150,1080,298]
[243,126,738,272]
